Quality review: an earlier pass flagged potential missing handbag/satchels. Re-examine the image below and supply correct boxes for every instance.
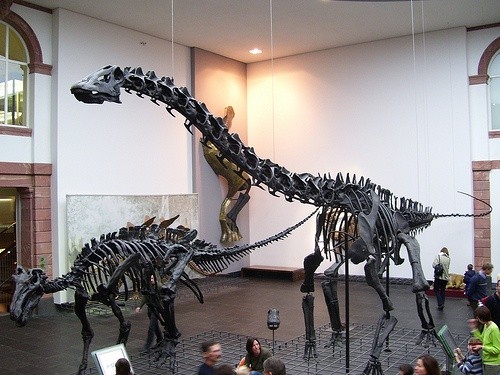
[435,264,443,276]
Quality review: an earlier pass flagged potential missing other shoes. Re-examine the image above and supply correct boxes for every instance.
[437,305,445,311]
[467,303,470,306]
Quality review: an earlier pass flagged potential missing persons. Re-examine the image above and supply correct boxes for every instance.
[263,357,286,375]
[216,364,237,375]
[454,337,483,375]
[197,339,221,375]
[467,306,500,375]
[136,275,163,352]
[237,337,272,371]
[399,364,414,375]
[415,355,440,375]
[432,248,450,310]
[463,263,500,331]
[115,358,133,375]
